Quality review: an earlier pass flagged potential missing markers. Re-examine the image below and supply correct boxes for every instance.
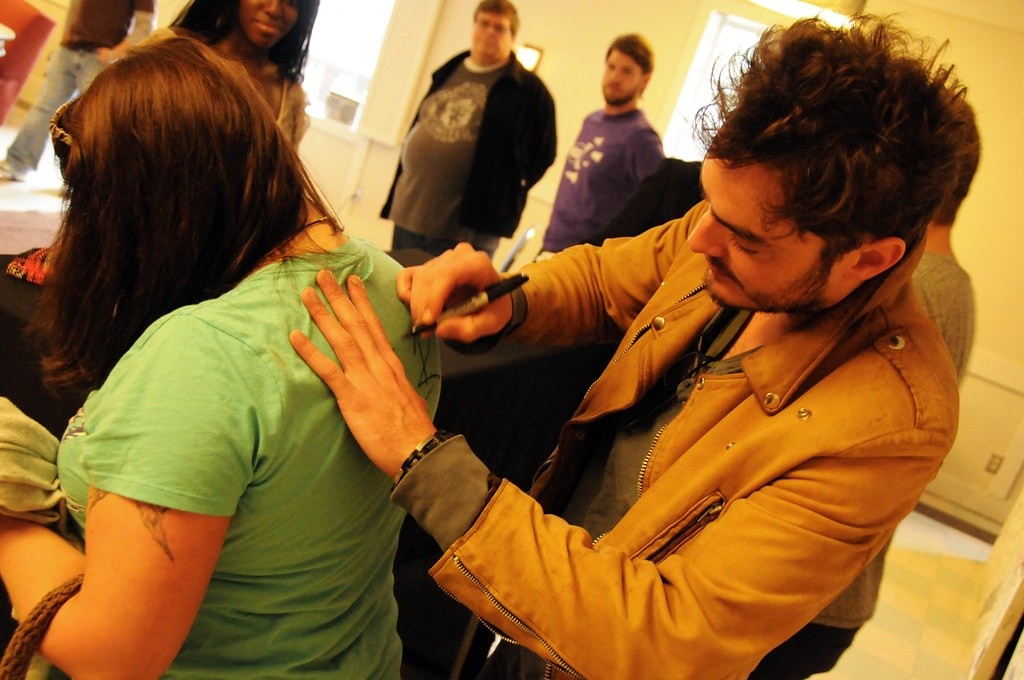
[401,271,531,344]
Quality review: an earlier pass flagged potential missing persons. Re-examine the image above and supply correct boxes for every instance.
[132,0,320,154]
[0,0,156,182]
[746,85,980,680]
[537,35,665,256]
[379,0,558,261]
[0,36,443,680]
[288,13,960,680]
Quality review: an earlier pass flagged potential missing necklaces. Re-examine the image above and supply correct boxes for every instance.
[294,218,328,232]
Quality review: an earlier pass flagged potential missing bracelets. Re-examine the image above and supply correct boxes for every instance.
[391,430,455,491]
